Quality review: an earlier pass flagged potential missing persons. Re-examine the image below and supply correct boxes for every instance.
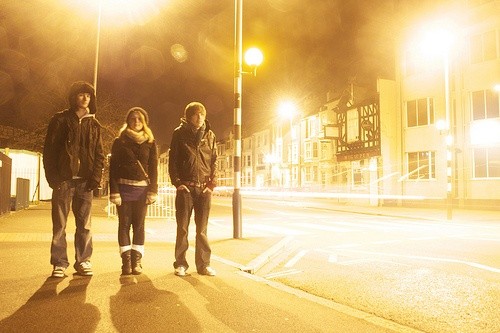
[168,102,219,276]
[43,82,103,277]
[109,108,158,274]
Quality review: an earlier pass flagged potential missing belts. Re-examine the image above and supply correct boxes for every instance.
[119,178,148,187]
[182,181,207,188]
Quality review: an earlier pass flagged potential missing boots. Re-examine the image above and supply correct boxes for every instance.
[120,245,133,275]
[132,244,144,274]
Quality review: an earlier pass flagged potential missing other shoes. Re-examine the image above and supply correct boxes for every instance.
[202,267,216,276]
[174,266,185,276]
[78,261,93,276]
[53,266,66,277]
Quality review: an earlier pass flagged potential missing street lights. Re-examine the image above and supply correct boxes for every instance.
[231,0,262,239]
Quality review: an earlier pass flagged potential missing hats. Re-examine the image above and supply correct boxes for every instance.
[186,102,206,123]
[126,106,149,126]
[69,81,97,114]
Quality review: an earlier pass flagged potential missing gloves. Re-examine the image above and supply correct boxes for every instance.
[146,192,157,205]
[110,193,122,206]
